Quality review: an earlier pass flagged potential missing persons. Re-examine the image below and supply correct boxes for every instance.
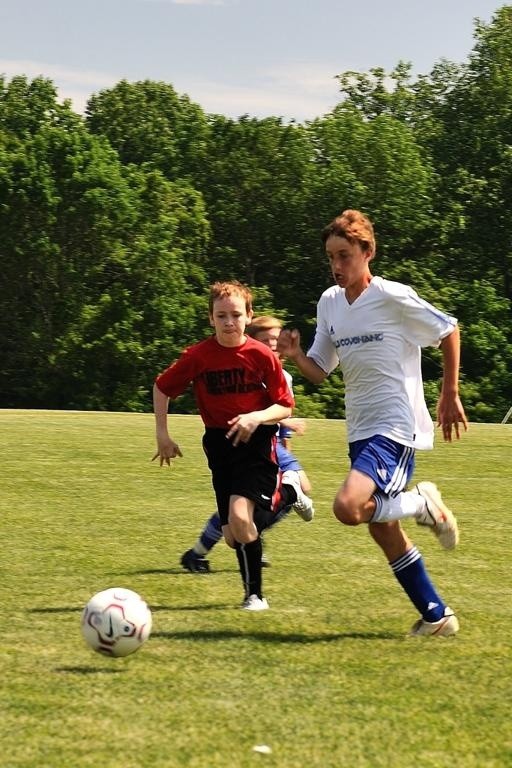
[152,279,295,612]
[180,315,312,574]
[276,208,468,636]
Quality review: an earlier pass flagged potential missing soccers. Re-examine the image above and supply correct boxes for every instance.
[80,587,153,657]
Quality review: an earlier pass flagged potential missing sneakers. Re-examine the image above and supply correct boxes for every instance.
[281,468,318,523]
[407,606,462,641]
[238,593,271,614]
[179,547,216,575]
[408,476,463,555]
[261,552,273,570]
